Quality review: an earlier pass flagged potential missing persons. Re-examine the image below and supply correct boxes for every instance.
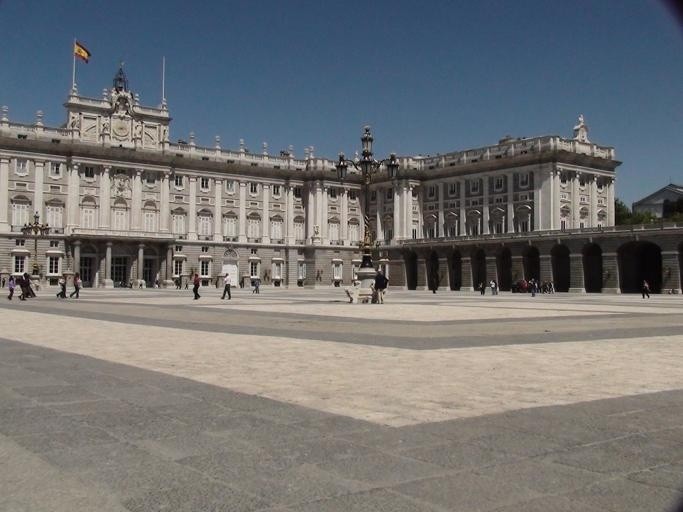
[55,274,67,298]
[479,281,485,295]
[492,280,498,295]
[191,274,201,299]
[69,272,79,298]
[489,280,496,295]
[252,279,259,293]
[5,272,37,300]
[641,279,650,299]
[59,277,64,298]
[220,273,231,299]
[527,278,553,297]
[374,269,388,303]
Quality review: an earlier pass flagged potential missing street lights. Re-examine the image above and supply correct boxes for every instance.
[20,210,51,292]
[335,125,400,304]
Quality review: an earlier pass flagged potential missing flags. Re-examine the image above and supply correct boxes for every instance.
[72,41,90,64]
